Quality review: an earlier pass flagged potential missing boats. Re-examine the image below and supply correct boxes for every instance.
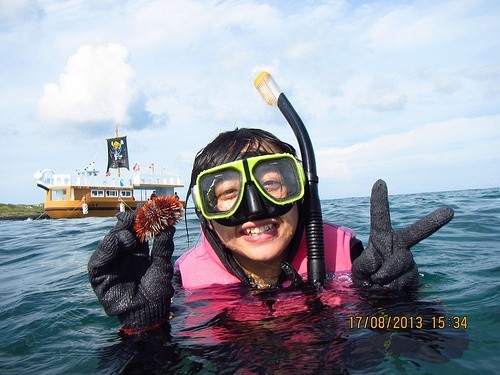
[35,125,187,218]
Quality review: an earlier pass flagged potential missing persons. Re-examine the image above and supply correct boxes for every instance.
[88,127,454,329]
[151,190,179,200]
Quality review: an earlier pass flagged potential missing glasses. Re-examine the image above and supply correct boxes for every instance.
[188,151,306,220]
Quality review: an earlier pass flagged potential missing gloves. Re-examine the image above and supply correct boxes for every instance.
[350,177,455,301]
[86,193,176,329]
